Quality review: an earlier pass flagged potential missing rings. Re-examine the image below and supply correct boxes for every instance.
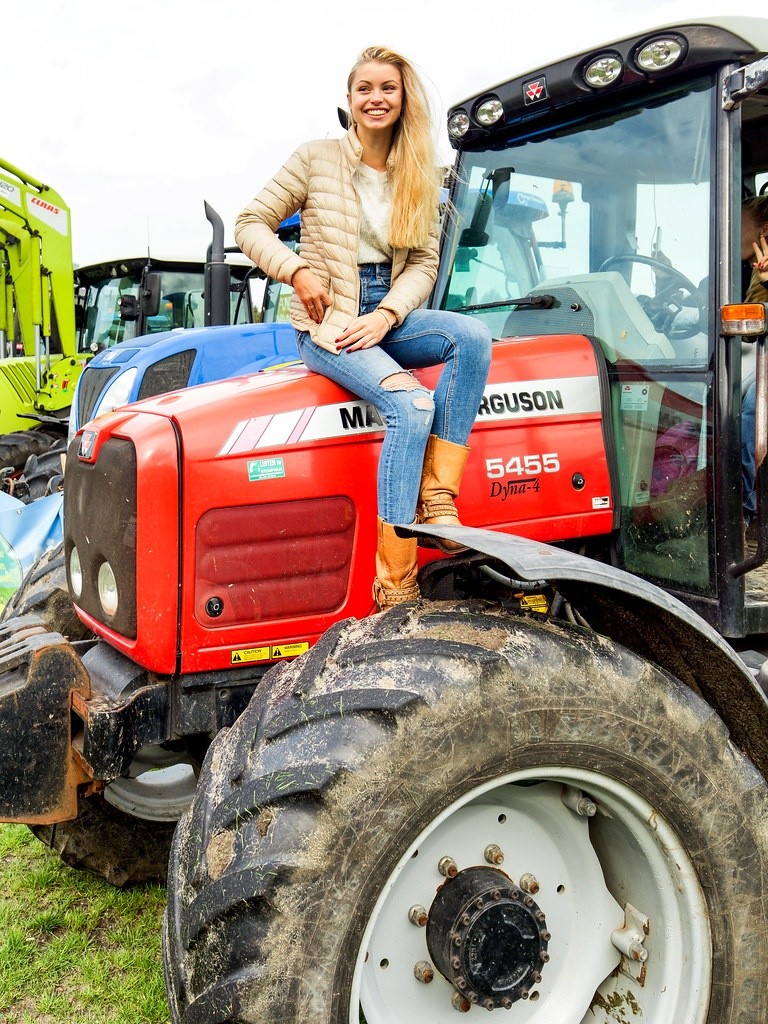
[307,305,315,308]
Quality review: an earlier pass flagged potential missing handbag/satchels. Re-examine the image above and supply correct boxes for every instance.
[649,418,712,524]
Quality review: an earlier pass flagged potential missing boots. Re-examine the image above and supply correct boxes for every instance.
[414,433,475,555]
[373,513,425,610]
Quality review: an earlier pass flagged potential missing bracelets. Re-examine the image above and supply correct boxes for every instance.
[374,309,392,331]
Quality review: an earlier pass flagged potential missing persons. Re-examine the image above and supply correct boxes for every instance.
[234,44,491,611]
[628,185,768,564]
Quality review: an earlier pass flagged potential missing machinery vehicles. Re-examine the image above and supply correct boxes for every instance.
[2,154,576,622]
[0,13,768,1024]
